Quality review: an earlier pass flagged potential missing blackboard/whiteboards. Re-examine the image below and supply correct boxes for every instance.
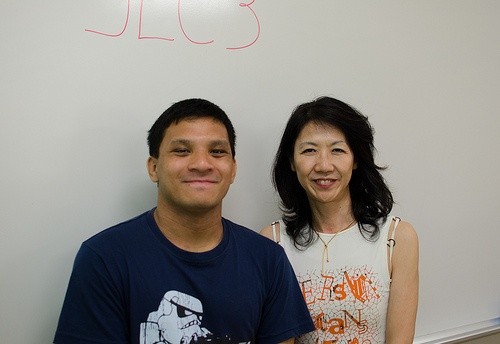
[0,0,500,343]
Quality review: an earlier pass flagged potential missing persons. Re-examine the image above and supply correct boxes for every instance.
[259,95,419,344]
[53,97,317,344]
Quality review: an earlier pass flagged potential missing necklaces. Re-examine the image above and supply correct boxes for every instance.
[311,218,356,275]
